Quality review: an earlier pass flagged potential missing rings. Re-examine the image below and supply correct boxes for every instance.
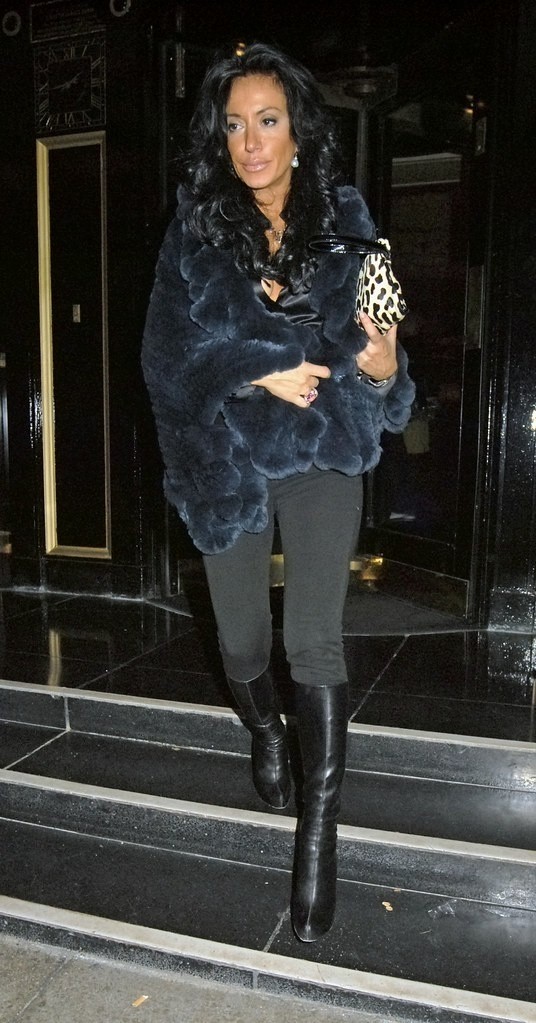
[305,390,316,403]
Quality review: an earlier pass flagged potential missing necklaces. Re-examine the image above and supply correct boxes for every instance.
[268,227,286,243]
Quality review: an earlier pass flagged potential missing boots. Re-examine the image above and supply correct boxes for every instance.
[290,676,349,943]
[225,650,292,809]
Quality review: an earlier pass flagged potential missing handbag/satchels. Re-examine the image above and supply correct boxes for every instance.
[307,234,408,335]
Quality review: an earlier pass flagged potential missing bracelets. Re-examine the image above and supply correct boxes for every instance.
[370,378,391,387]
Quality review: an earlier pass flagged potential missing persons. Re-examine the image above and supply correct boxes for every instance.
[139,44,414,943]
